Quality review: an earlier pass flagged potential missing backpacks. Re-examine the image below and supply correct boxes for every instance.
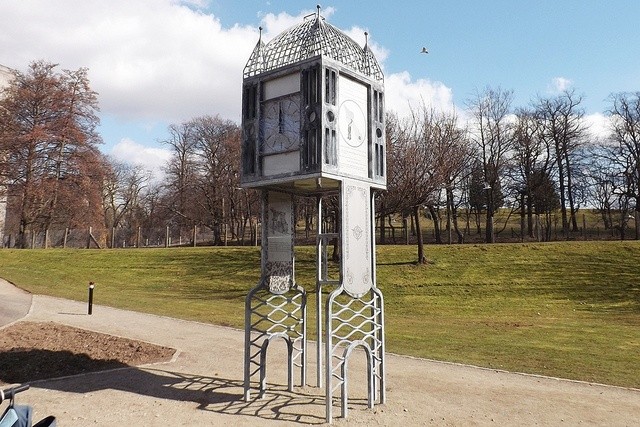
[0,388,56,427]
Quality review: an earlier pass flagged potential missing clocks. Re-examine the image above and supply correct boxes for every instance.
[259,93,301,154]
